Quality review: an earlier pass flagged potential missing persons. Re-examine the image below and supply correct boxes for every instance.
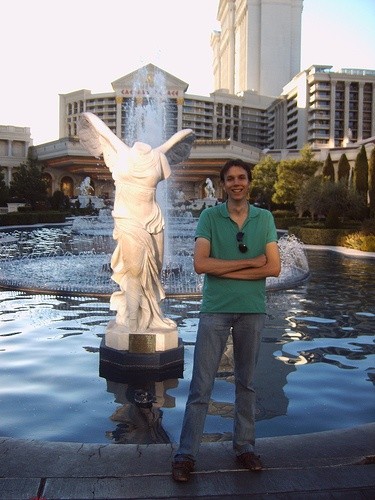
[171,161,282,483]
[84,111,195,329]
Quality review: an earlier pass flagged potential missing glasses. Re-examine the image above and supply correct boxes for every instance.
[237,232,248,253]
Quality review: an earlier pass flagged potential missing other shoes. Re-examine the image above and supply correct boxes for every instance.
[171,454,194,480]
[237,451,262,471]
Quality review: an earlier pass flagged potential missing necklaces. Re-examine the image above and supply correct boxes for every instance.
[226,200,248,217]
